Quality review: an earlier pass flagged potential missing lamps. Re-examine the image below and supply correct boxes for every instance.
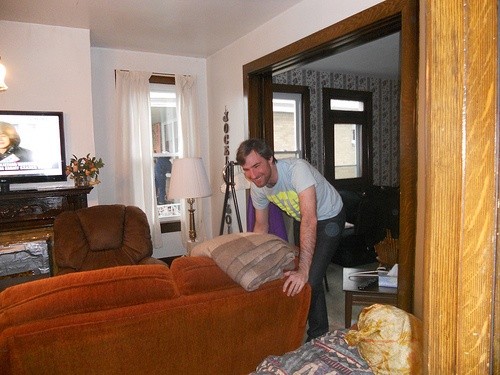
[0,61,8,91]
[166,156,213,257]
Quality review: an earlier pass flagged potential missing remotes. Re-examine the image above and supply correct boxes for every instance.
[358,278,378,290]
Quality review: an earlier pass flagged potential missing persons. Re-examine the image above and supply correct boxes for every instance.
[0,121,33,168]
[236,138,346,343]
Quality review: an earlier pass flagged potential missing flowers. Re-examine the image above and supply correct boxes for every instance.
[65,153,105,185]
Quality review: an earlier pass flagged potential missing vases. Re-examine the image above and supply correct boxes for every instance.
[74,174,92,187]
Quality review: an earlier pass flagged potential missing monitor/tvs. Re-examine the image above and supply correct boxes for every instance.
[0,110,68,193]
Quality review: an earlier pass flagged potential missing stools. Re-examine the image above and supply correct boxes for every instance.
[341,265,399,327]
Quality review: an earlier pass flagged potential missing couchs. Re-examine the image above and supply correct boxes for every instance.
[53,202,168,276]
[0,244,311,375]
